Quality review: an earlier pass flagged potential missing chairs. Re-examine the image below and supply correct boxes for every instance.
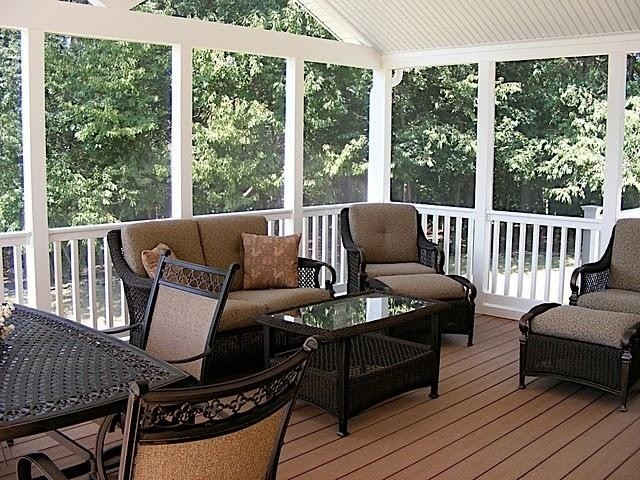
[569,219,640,315]
[340,204,445,295]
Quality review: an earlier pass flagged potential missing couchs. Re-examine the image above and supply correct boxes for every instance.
[107,213,336,372]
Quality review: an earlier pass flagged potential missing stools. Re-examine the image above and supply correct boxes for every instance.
[364,273,477,347]
[518,303,640,412]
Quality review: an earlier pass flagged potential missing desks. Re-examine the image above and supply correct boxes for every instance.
[1,293,193,439]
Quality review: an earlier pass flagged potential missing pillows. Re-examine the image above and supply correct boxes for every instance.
[141,242,188,287]
[240,230,302,290]
